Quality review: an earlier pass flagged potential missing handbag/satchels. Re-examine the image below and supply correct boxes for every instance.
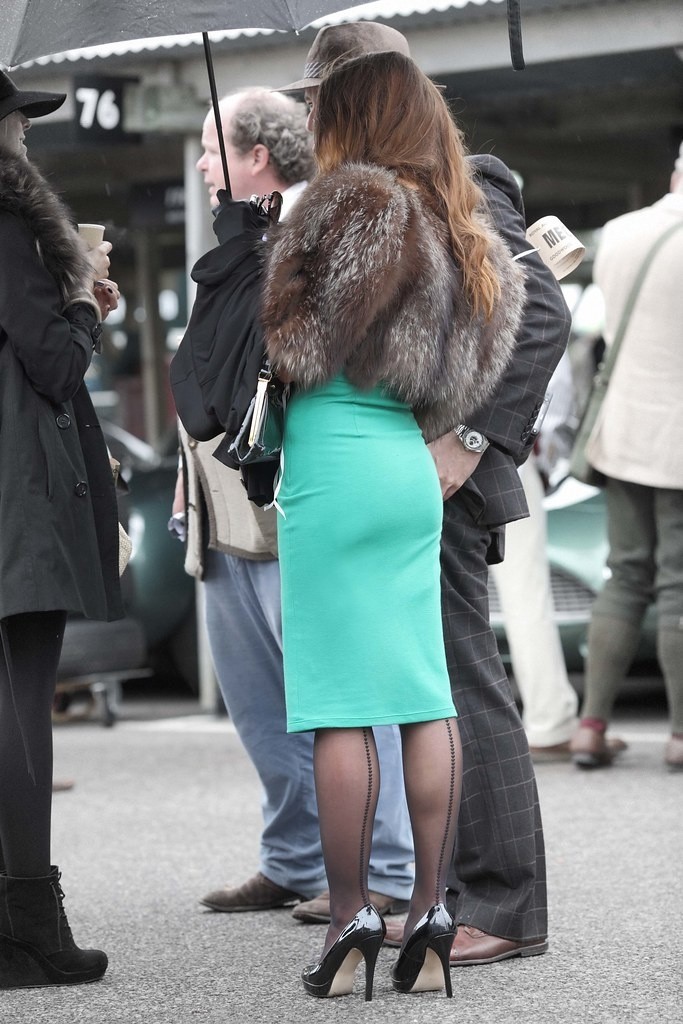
[228,369,287,514]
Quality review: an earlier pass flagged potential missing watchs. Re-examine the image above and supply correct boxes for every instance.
[455,424,489,453]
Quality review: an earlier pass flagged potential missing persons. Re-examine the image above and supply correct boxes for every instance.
[0,69,120,988]
[209,21,571,1002]
[159,86,414,922]
[571,141,683,769]
[495,348,627,764]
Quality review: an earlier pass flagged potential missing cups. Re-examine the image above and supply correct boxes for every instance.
[78,223,105,252]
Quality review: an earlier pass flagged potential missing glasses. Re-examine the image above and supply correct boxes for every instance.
[257,191,284,225]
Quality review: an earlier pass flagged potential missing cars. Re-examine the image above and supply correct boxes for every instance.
[43,410,234,716]
[473,485,664,679]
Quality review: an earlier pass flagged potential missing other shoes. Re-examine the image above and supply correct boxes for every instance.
[290,889,408,921]
[574,753,612,766]
[528,736,627,763]
[664,738,683,763]
[198,873,309,912]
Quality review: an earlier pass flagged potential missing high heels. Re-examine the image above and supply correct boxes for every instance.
[388,903,459,999]
[299,901,386,1002]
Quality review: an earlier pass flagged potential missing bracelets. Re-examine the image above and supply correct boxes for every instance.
[61,298,101,323]
[71,287,98,302]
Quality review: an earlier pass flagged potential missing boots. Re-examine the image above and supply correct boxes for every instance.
[0,865,107,988]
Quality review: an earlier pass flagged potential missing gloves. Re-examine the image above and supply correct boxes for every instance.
[210,189,269,244]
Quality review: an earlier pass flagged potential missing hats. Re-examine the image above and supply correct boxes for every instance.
[270,22,446,103]
[0,70,66,123]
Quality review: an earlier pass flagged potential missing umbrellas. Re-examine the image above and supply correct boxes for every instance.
[0,0,386,200]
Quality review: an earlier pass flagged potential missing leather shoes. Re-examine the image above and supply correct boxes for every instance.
[379,912,408,947]
[449,923,548,964]
